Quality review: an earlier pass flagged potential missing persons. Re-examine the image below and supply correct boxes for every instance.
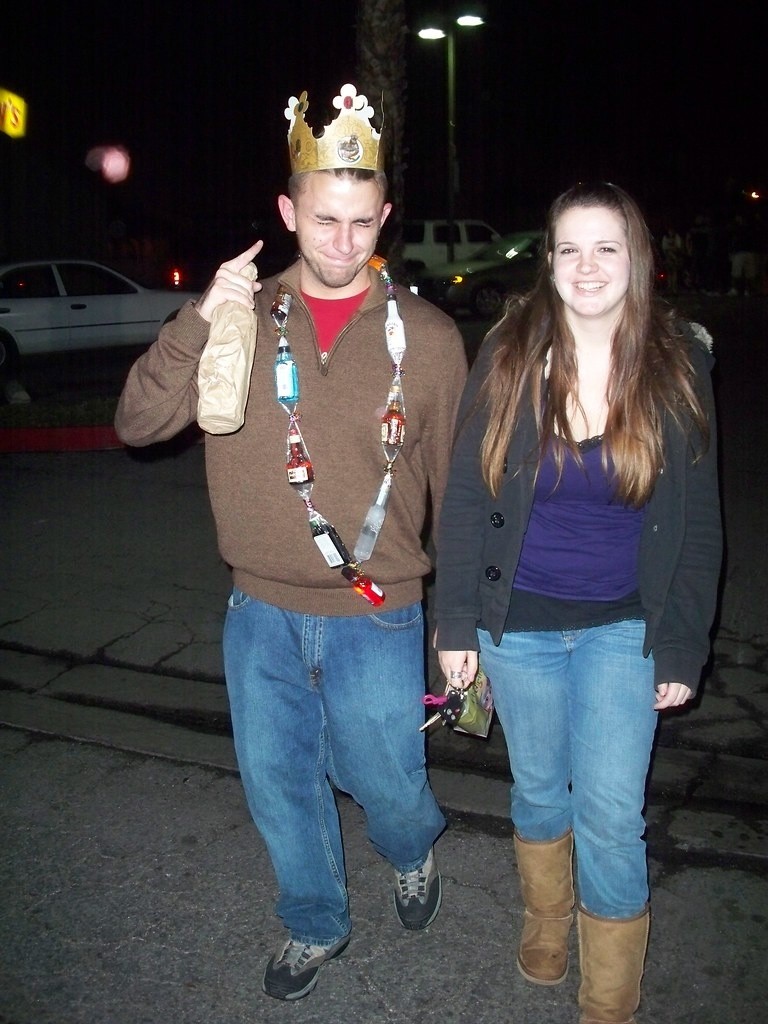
[435,180,725,1024]
[111,83,472,1000]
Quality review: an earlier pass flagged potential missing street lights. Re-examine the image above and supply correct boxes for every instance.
[415,10,486,264]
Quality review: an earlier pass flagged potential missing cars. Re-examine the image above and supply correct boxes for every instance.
[0,257,204,383]
[411,230,544,321]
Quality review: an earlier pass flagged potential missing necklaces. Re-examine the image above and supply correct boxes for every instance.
[270,254,405,607]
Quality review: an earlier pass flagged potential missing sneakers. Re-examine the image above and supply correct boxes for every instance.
[260,928,351,1000]
[393,842,440,929]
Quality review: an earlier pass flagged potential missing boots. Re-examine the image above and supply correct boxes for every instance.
[514,828,574,985]
[576,896,651,1024]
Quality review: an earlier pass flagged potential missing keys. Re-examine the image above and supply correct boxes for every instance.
[419,690,464,732]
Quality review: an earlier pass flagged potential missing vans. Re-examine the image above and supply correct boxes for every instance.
[400,219,505,275]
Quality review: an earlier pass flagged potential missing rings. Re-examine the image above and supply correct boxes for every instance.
[451,671,461,678]
